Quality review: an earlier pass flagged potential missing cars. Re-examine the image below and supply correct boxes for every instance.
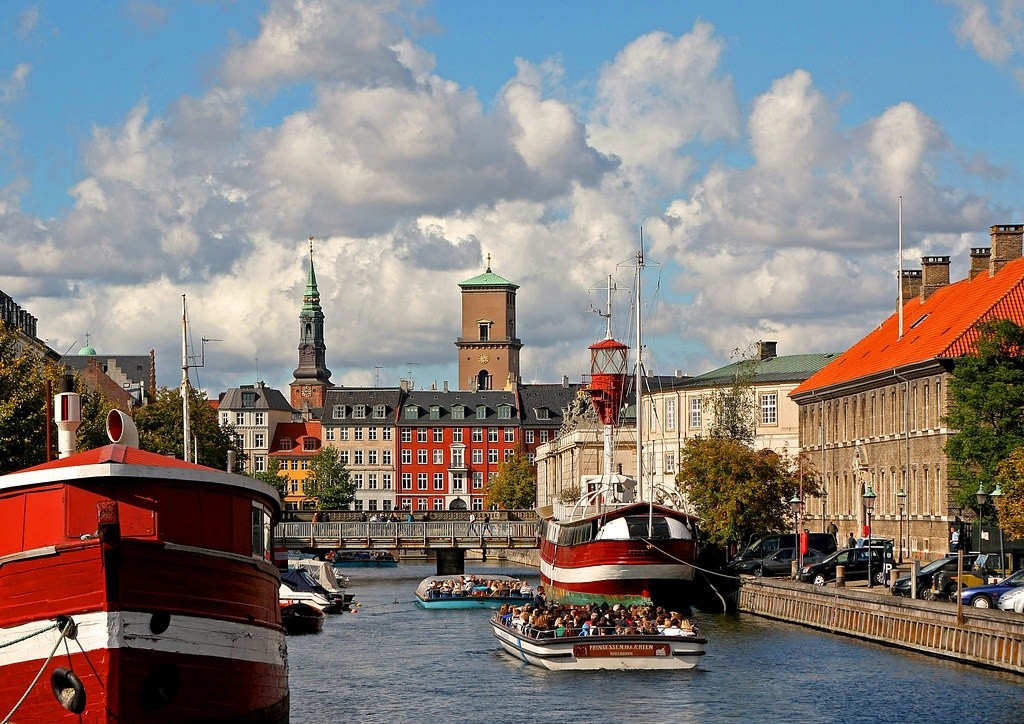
[853,537,893,558]
[733,547,829,576]
[891,552,1024,614]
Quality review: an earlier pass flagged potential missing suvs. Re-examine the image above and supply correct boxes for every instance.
[730,533,837,561]
[794,547,897,586]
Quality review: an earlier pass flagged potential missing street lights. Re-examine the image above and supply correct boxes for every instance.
[789,491,803,578]
[895,488,907,564]
[974,481,988,552]
[990,481,1006,579]
[819,486,829,533]
[863,482,876,587]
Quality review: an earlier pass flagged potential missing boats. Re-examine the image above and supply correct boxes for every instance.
[488,600,707,673]
[333,550,399,567]
[0,295,290,723]
[274,548,354,630]
[412,573,536,609]
[540,227,702,610]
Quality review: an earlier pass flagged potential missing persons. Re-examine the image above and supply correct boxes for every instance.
[422,511,431,536]
[359,511,401,536]
[950,527,960,551]
[827,521,838,543]
[466,512,478,536]
[426,575,534,599]
[311,511,331,536]
[408,510,415,536]
[534,586,547,608]
[325,551,394,561]
[848,532,857,560]
[495,600,696,638]
[481,513,492,536]
